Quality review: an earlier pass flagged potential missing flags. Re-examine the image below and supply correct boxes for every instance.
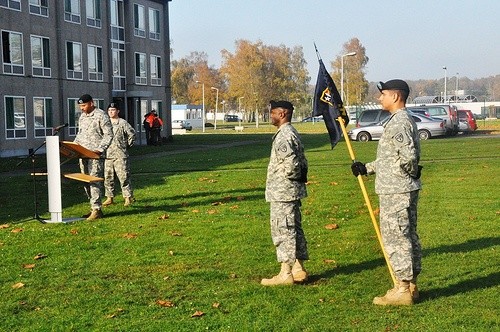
[313,60,349,152]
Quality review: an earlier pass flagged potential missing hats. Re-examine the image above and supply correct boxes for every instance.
[377,80,409,93]
[269,100,294,111]
[108,102,120,110]
[78,94,92,104]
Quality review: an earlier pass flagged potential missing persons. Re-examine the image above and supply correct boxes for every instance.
[350,79,423,306]
[72,94,114,221]
[102,103,137,206]
[261,101,309,286]
[143,110,162,145]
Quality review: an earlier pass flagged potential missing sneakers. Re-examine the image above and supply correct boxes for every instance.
[102,197,113,205]
[87,209,103,221]
[124,197,132,207]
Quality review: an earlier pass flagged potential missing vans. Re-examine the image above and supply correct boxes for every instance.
[224,115,243,122]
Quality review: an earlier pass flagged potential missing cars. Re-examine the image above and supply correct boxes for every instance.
[302,116,321,122]
[171,119,192,131]
[349,111,448,142]
[347,104,478,136]
[14,112,44,128]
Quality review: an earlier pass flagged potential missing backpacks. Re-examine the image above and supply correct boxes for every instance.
[153,118,160,128]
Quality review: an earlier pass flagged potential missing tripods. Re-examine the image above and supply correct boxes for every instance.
[12,129,60,225]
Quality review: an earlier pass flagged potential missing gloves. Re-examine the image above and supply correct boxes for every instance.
[300,169,307,183]
[351,162,367,177]
[412,165,423,180]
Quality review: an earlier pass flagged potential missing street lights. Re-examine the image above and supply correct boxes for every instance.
[255,98,259,128]
[455,72,460,102]
[442,66,447,103]
[211,86,218,130]
[238,96,245,128]
[222,100,226,121]
[196,80,206,133]
[340,51,357,104]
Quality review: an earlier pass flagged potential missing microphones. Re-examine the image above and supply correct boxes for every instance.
[55,123,69,130]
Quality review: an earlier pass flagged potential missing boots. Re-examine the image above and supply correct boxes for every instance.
[291,261,308,282]
[260,263,294,285]
[372,280,414,305]
[386,274,419,300]
